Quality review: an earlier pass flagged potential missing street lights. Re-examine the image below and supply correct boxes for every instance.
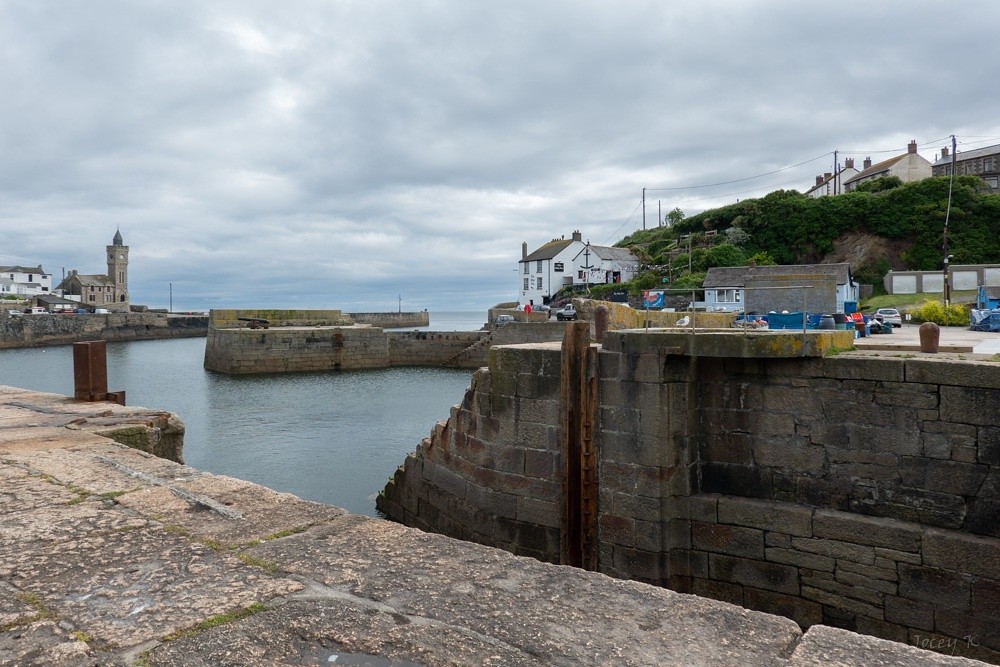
[943,254,954,306]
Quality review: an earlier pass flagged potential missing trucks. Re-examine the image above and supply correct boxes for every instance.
[95,309,113,314]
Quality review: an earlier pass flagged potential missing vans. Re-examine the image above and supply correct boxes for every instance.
[6,309,24,315]
[27,307,49,314]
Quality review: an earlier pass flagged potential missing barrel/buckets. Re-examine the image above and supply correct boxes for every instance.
[736,300,893,339]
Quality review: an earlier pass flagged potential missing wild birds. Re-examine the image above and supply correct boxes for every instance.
[676,316,691,328]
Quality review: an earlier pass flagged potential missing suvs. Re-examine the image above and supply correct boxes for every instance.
[556,303,576,321]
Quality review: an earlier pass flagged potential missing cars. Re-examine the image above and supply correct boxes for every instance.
[495,314,514,327]
[871,308,902,328]
[52,309,90,313]
